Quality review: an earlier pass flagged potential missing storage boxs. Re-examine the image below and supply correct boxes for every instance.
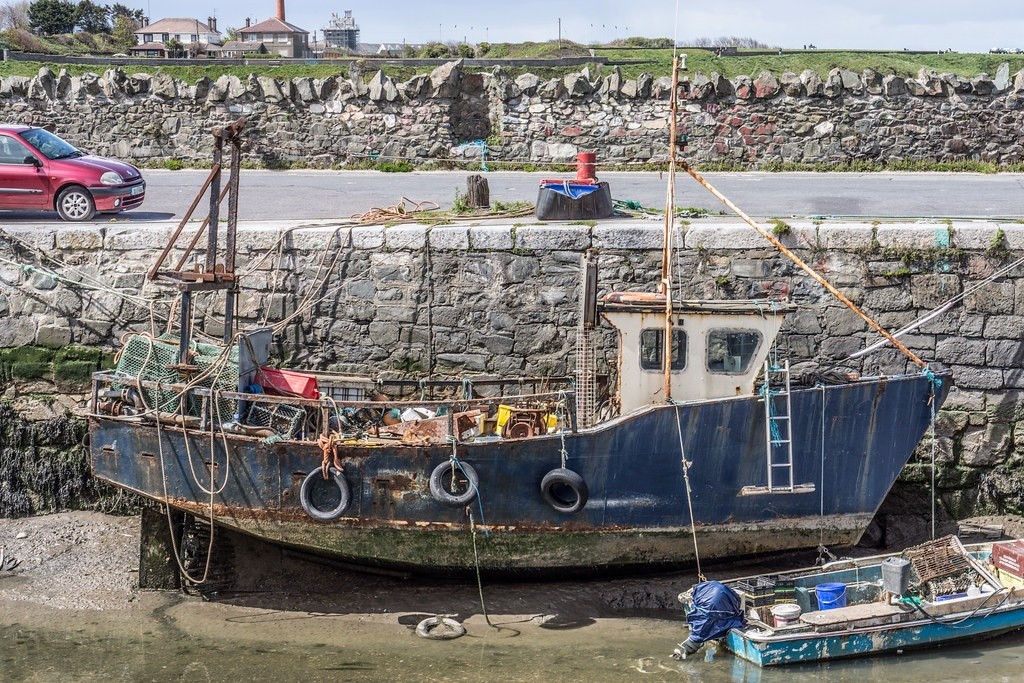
[992,540,1024,578]
[775,599,797,604]
[761,575,796,590]
[774,590,795,599]
[736,578,774,596]
[745,593,774,607]
[254,367,319,400]
[998,569,1024,588]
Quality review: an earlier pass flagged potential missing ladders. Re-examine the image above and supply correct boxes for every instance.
[765,359,794,494]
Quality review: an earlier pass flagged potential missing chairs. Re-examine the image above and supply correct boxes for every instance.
[0,141,13,163]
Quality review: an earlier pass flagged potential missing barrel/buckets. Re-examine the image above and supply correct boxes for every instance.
[770,604,801,628]
[815,582,846,611]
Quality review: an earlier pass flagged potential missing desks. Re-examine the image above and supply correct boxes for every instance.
[799,601,913,631]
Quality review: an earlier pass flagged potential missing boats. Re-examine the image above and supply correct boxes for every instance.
[671,537,1024,669]
[89,55,952,572]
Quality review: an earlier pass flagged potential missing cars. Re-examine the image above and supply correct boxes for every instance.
[0,123,146,221]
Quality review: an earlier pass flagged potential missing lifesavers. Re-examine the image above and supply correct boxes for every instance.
[539,466,588,514]
[299,464,352,523]
[428,457,481,508]
